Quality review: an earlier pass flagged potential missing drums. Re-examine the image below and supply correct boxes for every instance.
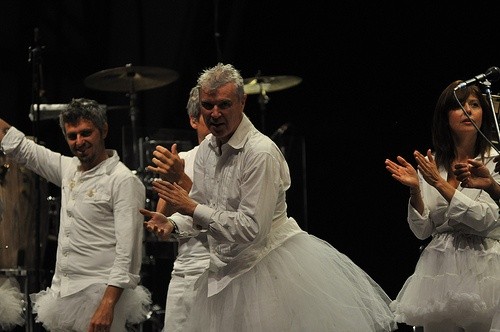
[0,137,48,276]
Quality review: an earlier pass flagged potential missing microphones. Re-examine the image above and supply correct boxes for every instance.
[454,67,500,91]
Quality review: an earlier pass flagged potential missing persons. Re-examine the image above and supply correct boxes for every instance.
[385,80,500,332]
[453,154,500,223]
[0,98,151,332]
[138,62,397,332]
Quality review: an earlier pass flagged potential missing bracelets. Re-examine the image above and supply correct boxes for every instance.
[170,219,179,234]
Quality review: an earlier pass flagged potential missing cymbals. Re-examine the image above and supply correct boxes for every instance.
[237,76,303,95]
[82,66,176,91]
